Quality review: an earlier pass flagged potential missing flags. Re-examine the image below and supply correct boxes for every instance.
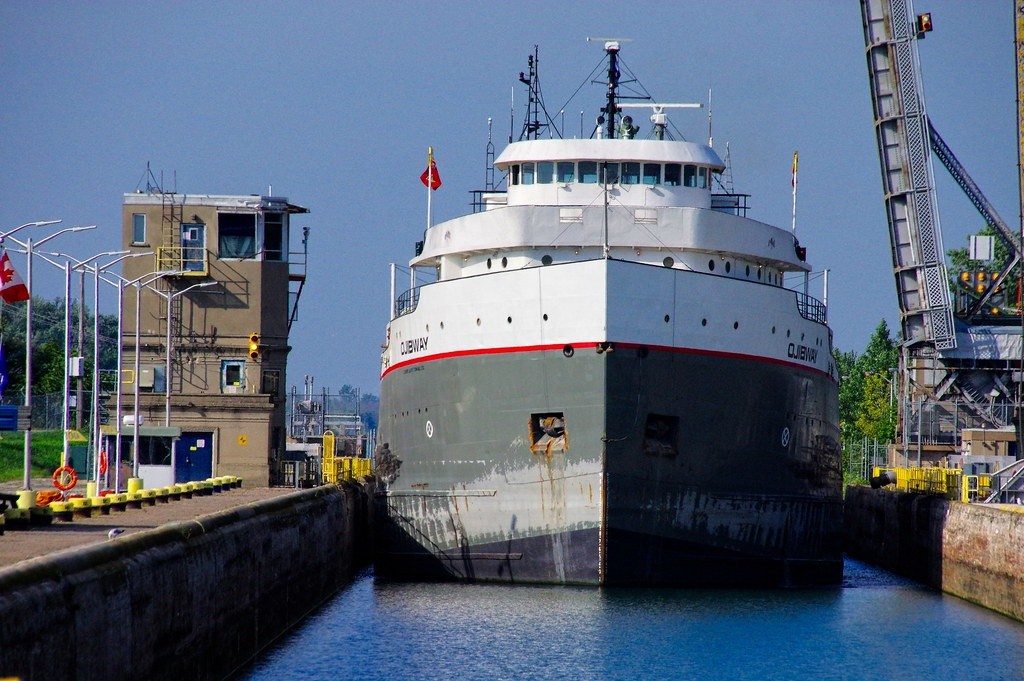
[0,244,30,303]
[420,156,442,190]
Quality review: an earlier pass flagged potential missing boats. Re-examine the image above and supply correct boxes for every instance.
[371,36,844,591]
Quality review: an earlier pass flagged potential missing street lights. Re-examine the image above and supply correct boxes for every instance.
[74,269,177,495]
[0,217,97,507]
[96,268,193,493]
[134,280,217,426]
[1,249,133,501]
[39,250,154,502]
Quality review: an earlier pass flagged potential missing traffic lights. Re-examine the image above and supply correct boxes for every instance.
[249,332,260,361]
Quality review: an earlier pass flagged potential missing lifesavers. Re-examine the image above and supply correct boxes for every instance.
[100,452,107,474]
[53,467,77,491]
